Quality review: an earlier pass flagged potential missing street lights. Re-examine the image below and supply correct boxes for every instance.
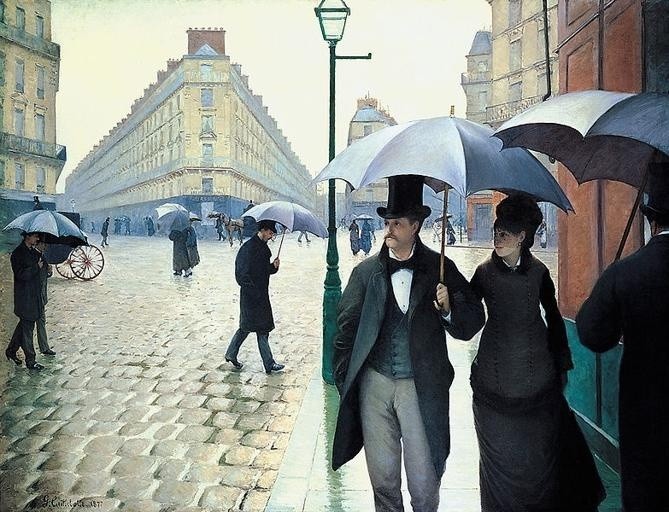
[70,199,76,213]
[315,0,350,386]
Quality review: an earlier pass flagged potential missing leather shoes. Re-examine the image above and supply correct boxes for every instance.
[6,349,56,370]
[271,363,284,370]
[226,357,239,366]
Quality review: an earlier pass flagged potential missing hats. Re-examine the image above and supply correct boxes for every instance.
[262,220,277,234]
[640,164,669,221]
[377,175,431,220]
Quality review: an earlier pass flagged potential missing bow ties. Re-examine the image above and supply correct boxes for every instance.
[388,258,412,274]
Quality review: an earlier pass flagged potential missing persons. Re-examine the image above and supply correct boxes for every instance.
[330,215,484,512]
[538,218,547,248]
[577,213,669,512]
[446,220,456,245]
[169,228,193,277]
[225,222,286,375]
[5,232,44,370]
[452,195,605,512]
[34,237,56,355]
[33,201,44,211]
[101,219,110,247]
[298,230,311,243]
[148,217,155,237]
[184,220,200,269]
[361,219,376,255]
[338,215,383,230]
[431,219,442,243]
[113,215,131,235]
[215,216,226,241]
[144,216,149,237]
[349,219,361,256]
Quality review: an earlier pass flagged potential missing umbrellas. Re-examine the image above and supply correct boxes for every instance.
[155,202,190,233]
[356,214,373,220]
[3,208,85,259]
[20,229,90,277]
[190,212,202,226]
[242,199,329,258]
[438,213,452,219]
[490,88,668,261]
[207,212,224,219]
[310,105,575,310]
